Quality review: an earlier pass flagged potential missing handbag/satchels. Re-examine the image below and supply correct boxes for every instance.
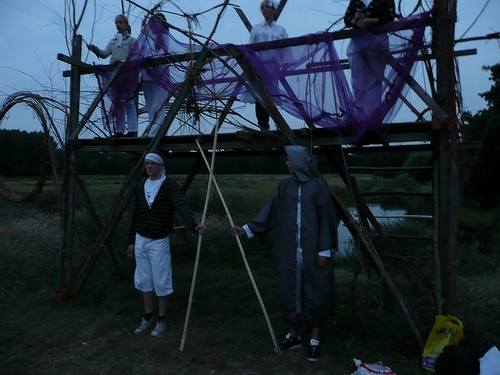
[416,314,468,372]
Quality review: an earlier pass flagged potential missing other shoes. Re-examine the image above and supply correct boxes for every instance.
[112,132,124,138]
[151,321,167,336]
[134,320,153,333]
[123,132,137,138]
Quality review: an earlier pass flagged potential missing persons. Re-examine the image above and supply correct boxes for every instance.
[230,145,337,363]
[247,0,296,132]
[344,0,396,125]
[87,15,140,137]
[141,13,170,137]
[127,149,207,337]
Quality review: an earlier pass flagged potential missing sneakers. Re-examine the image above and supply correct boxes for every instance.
[273,330,302,352]
[306,338,321,361]
